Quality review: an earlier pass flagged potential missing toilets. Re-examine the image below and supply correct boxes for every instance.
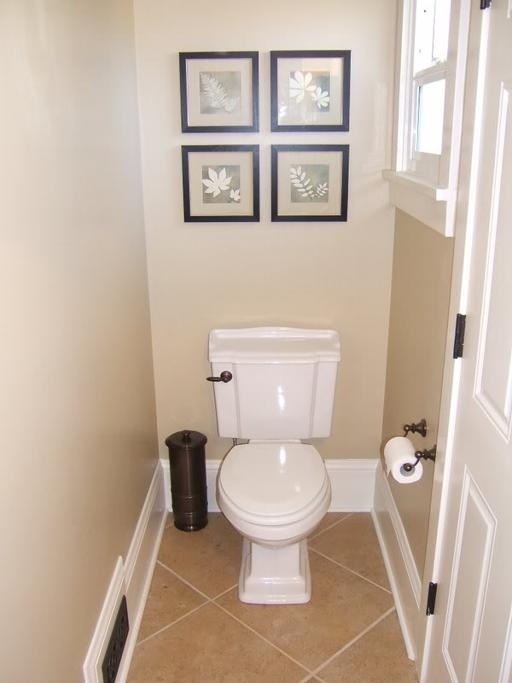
[206,327,340,604]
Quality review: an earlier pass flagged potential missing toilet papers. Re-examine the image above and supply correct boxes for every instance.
[382,436,422,485]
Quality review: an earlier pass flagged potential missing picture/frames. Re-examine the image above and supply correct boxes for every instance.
[179,51,352,223]
